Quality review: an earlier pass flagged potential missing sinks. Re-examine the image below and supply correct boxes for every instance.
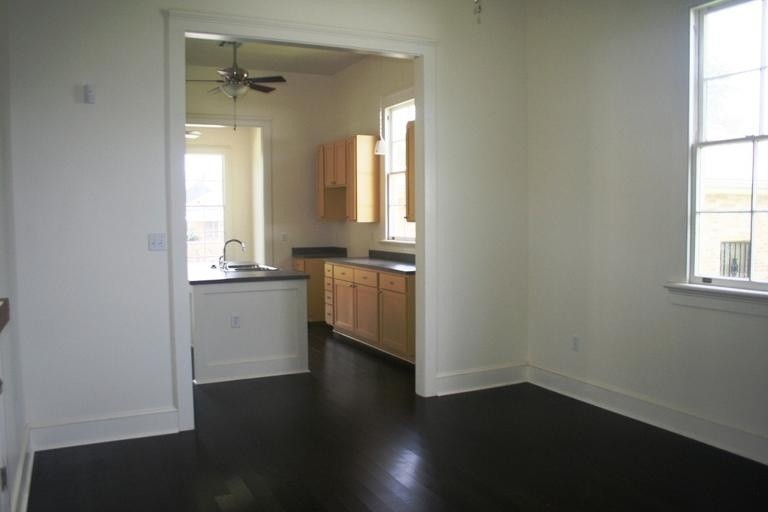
[228,264,260,268]
[235,267,278,273]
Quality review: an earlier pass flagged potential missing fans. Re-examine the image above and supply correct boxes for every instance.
[185,36,288,97]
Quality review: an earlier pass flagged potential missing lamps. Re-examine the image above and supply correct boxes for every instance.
[373,129,390,157]
[215,77,251,99]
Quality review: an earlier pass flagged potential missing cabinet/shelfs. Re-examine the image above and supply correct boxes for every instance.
[322,261,334,327]
[354,267,413,361]
[334,264,353,345]
[313,140,347,220]
[347,133,381,224]
[324,140,347,186]
[403,119,417,224]
[291,252,345,321]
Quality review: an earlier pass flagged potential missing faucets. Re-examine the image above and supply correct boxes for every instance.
[223,239,246,261]
